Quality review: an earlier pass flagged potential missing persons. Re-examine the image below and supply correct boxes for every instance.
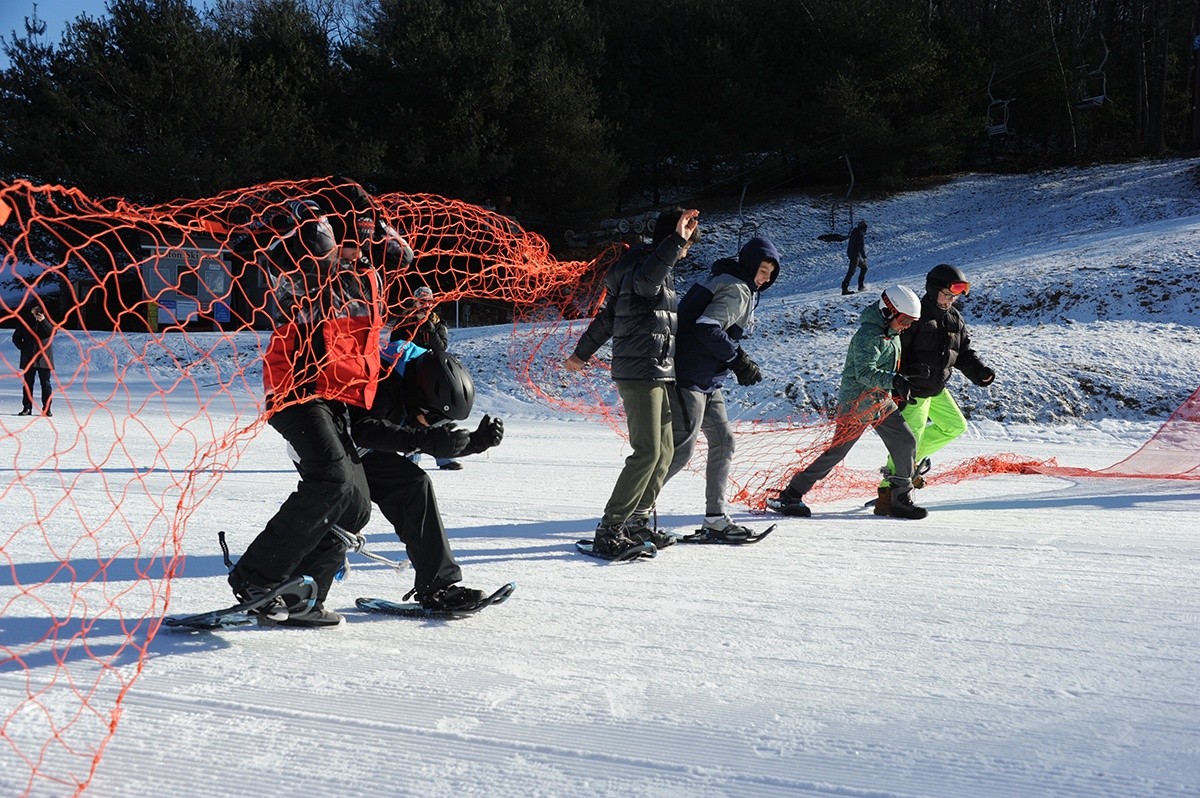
[12,297,57,418]
[660,235,782,542]
[392,284,461,471]
[778,282,927,521]
[277,340,484,622]
[230,176,416,625]
[840,221,869,296]
[873,264,995,517]
[561,205,701,561]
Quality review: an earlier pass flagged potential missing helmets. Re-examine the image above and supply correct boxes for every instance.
[879,285,922,334]
[285,198,320,220]
[926,262,970,311]
[410,349,476,420]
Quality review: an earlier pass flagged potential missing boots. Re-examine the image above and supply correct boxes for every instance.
[777,486,811,513]
[890,484,927,519]
[858,280,868,291]
[841,284,855,295]
[874,486,890,515]
[913,463,924,489]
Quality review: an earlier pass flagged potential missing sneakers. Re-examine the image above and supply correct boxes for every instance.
[626,528,664,547]
[419,584,485,612]
[234,578,287,622]
[708,517,745,539]
[593,525,635,556]
[292,611,340,629]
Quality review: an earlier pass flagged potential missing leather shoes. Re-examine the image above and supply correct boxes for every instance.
[17,407,31,416]
[42,408,53,416]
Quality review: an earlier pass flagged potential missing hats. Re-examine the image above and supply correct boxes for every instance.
[332,194,377,248]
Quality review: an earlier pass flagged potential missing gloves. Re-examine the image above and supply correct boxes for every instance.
[418,422,470,459]
[728,347,762,387]
[469,413,504,454]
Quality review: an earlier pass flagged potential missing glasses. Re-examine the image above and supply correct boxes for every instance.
[417,408,442,424]
[949,280,969,297]
[895,309,916,327]
[940,289,958,299]
